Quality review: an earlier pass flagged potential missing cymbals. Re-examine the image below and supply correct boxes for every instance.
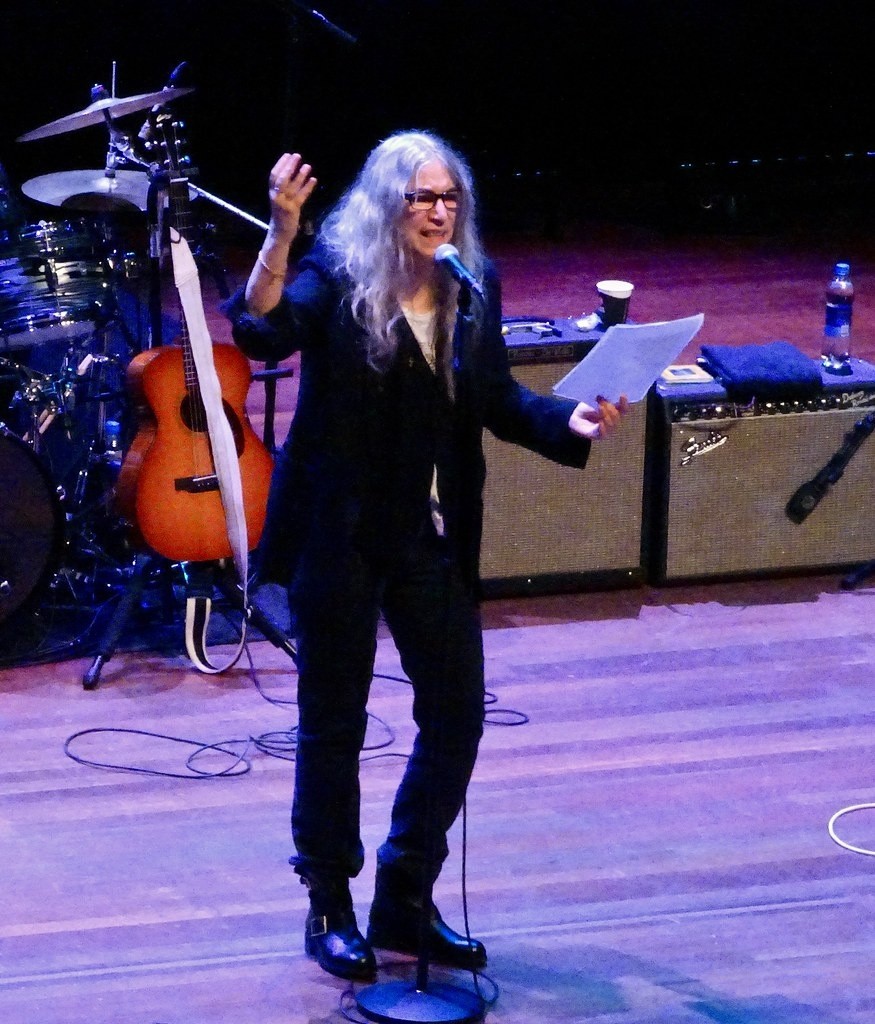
[19,165,201,217]
[12,85,200,147]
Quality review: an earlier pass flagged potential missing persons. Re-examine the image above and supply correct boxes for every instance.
[218,130,630,982]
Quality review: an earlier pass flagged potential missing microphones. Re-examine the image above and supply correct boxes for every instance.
[138,62,188,139]
[434,243,483,297]
[785,451,842,525]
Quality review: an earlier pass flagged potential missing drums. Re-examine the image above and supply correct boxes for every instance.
[3,257,115,358]
[6,217,115,261]
[1,393,75,641]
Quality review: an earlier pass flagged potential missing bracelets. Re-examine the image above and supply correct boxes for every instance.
[257,250,287,277]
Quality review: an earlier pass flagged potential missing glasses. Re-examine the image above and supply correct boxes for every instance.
[404,186,464,210]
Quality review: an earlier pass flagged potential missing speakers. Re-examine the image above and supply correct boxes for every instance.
[651,355,875,588]
[478,315,648,597]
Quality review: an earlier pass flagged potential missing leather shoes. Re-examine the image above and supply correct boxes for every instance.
[366,875,488,969]
[304,888,376,979]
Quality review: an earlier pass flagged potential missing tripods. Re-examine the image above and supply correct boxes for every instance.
[31,108,300,691]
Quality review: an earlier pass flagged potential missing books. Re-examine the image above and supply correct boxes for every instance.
[661,363,714,384]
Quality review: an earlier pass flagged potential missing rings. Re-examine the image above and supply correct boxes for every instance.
[273,186,283,193]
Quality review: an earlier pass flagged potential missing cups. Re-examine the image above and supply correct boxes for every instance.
[595,280,634,329]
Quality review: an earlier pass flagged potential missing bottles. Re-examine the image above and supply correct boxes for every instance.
[100,420,123,474]
[821,263,855,369]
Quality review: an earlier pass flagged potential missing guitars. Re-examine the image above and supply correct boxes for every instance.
[112,99,274,567]
